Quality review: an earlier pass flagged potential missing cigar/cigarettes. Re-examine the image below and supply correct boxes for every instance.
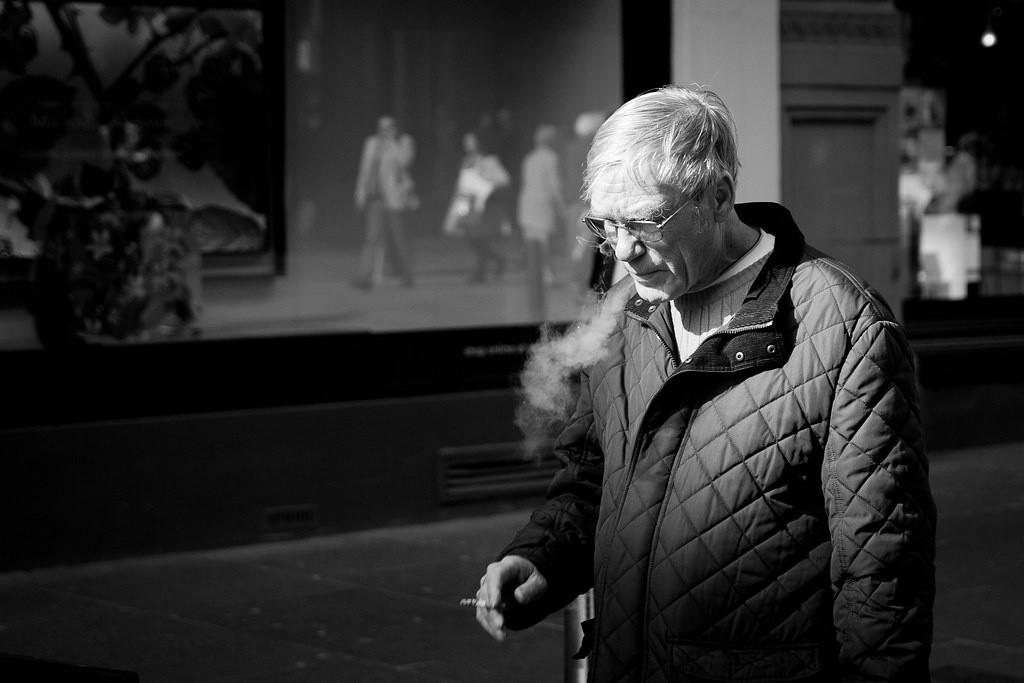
[461,598,505,608]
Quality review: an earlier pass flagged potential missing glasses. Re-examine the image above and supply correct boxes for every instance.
[582,187,707,243]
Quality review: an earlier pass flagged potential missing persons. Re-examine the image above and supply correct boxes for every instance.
[355,117,420,290]
[520,125,567,322]
[463,135,511,282]
[477,88,939,682]
